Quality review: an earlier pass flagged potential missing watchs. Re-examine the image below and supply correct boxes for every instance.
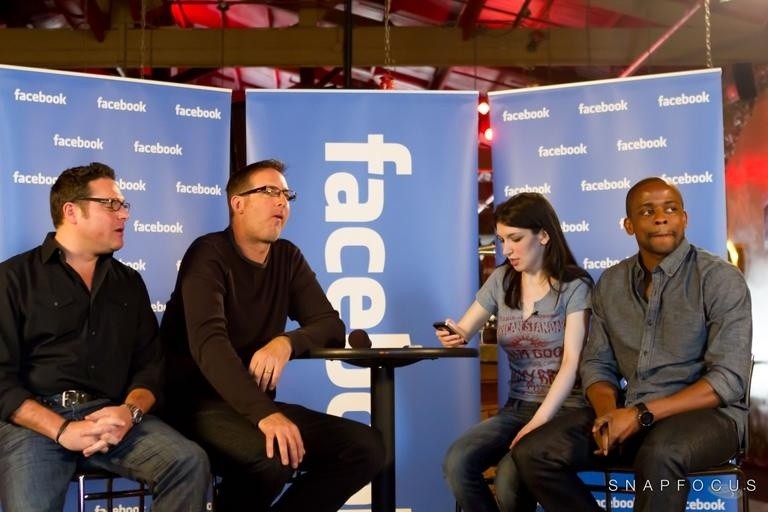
[635,402,654,429]
[124,401,143,425]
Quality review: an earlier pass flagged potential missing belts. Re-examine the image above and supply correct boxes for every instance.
[34,387,110,411]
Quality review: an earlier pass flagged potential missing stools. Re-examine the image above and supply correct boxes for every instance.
[69,465,153,512]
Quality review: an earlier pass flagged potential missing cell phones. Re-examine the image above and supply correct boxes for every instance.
[433,322,469,345]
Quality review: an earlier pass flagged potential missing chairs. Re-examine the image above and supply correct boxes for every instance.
[587,351,758,512]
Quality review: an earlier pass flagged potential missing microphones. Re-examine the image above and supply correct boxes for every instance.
[348,329,372,348]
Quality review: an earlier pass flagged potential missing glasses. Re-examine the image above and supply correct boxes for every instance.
[236,183,299,203]
[69,193,132,213]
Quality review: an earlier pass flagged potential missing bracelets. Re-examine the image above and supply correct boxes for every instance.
[55,419,73,443]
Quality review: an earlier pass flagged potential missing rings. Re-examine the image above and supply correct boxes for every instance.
[262,370,272,376]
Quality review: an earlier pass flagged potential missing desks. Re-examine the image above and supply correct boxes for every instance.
[291,346,479,512]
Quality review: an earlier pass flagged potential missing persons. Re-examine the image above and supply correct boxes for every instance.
[154,158,386,512]
[435,192,599,512]
[0,162,212,512]
[512,176,752,512]
